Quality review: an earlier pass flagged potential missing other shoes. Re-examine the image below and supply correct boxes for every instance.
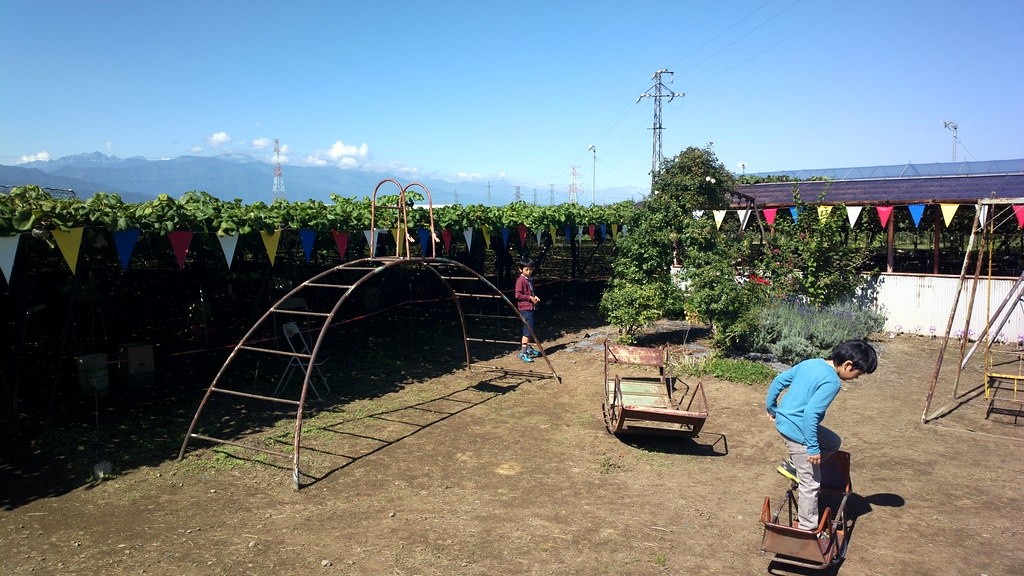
[818,531,829,542]
[776,458,801,483]
[527,348,540,356]
[518,352,534,362]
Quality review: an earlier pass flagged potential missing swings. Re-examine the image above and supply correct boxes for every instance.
[982,205,1024,406]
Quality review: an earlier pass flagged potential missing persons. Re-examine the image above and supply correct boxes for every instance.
[515,256,541,362]
[766,340,878,533]
